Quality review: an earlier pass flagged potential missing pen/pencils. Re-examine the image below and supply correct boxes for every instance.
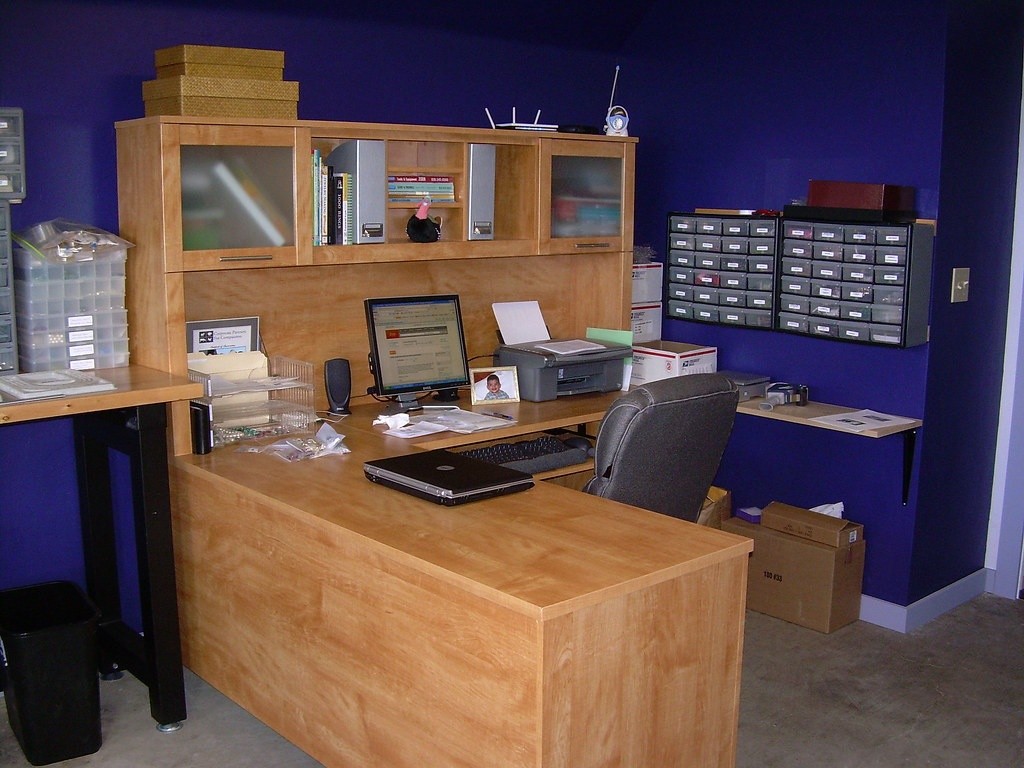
[481,412,514,421]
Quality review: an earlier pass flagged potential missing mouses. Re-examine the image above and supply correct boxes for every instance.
[567,439,595,458]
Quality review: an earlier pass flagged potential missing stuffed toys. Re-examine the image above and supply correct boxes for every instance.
[406,197,444,242]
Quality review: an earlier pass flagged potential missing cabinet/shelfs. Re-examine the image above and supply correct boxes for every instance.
[113,115,935,768]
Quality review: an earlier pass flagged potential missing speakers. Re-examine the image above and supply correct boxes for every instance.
[324,358,352,416]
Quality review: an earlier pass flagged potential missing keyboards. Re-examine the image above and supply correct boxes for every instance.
[457,436,586,474]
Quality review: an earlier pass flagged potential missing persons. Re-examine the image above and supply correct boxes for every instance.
[485,375,509,400]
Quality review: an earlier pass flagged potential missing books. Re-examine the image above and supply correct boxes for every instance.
[309,139,456,247]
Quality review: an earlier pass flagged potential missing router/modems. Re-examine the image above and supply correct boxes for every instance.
[485,107,558,132]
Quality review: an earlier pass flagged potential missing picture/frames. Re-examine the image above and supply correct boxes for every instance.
[468,366,522,407]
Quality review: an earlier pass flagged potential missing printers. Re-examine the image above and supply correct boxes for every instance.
[493,325,633,402]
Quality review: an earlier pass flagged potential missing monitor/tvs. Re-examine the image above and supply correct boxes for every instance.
[363,294,471,417]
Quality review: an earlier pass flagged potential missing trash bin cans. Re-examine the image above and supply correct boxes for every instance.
[1,598,104,765]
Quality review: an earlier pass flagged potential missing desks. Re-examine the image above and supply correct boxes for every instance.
[0,362,204,732]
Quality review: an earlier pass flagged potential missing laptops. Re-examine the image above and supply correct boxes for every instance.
[364,449,536,506]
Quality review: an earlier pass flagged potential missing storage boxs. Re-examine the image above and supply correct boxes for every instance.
[0,105,25,374]
[12,247,130,373]
[630,340,718,387]
[696,485,732,530]
[631,301,663,343]
[552,192,624,237]
[761,501,865,549]
[807,178,917,212]
[720,517,866,634]
[628,261,663,303]
